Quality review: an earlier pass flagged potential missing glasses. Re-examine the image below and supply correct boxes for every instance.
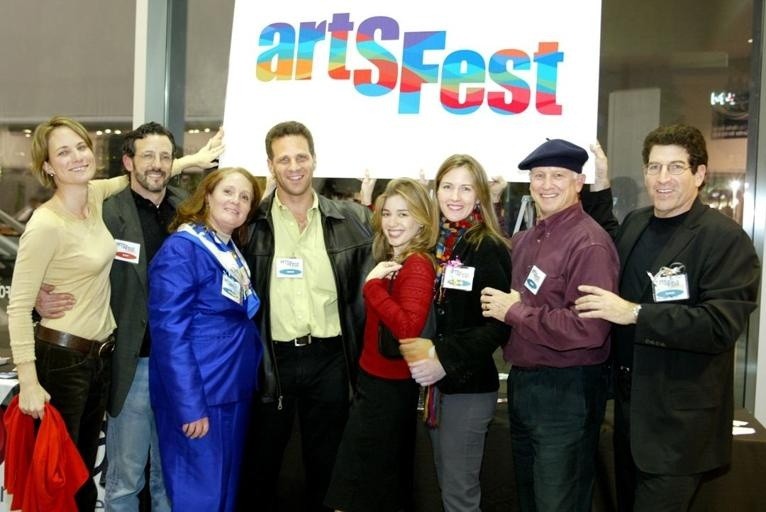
[132,151,172,163]
[642,160,692,176]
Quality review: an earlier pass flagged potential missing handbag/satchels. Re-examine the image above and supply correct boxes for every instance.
[377,253,438,360]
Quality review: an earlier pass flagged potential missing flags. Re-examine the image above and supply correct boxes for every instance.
[220,1,605,186]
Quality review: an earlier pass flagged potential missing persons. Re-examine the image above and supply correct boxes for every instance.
[31,120,194,511]
[229,122,378,512]
[318,177,435,512]
[480,138,620,512]
[590,125,761,512]
[147,168,265,512]
[409,154,511,511]
[6,113,221,511]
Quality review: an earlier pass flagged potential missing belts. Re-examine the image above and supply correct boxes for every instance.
[35,325,116,358]
[271,335,342,350]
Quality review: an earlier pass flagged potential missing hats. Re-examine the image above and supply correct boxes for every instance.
[518,139,589,174]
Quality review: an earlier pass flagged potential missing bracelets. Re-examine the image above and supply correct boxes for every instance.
[635,301,643,324]
[485,303,487,308]
